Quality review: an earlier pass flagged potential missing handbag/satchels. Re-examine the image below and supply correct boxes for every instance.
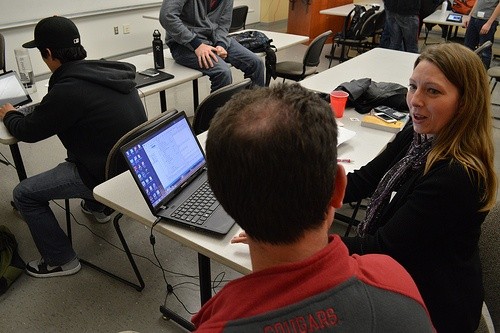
[451,0,476,15]
[227,31,278,53]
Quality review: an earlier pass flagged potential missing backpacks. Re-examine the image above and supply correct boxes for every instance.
[0,224,26,295]
[344,4,375,41]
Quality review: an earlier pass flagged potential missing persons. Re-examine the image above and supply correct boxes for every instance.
[159,0,265,94]
[232,43,498,333]
[0,15,148,277]
[186,81,438,333]
[377,0,500,71]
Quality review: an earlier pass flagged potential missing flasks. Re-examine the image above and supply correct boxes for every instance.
[153,29,165,69]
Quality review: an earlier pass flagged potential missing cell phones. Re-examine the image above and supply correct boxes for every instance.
[140,69,159,77]
[375,105,405,119]
[374,112,397,122]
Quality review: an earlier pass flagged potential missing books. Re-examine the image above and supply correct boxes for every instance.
[361,114,404,133]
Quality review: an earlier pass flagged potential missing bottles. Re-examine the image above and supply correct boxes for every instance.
[441,1,447,14]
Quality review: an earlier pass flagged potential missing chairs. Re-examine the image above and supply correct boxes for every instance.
[1,1,500,332]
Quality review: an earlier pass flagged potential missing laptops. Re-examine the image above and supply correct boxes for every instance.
[120,110,236,235]
[0,70,42,116]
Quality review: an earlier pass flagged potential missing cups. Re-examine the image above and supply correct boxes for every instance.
[14,47,34,87]
[330,90,349,119]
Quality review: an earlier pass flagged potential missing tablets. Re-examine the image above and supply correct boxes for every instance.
[447,14,462,22]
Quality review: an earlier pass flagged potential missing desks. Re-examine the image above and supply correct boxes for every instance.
[93,103,395,332]
[299,46,438,140]
[423,4,469,41]
[150,28,309,117]
[319,0,386,52]
[0,54,199,208]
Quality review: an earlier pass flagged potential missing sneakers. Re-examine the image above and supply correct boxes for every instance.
[80,199,111,223]
[25,256,82,278]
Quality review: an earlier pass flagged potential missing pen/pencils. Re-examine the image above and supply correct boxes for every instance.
[337,157,351,163]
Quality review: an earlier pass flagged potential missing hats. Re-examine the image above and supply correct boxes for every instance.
[22,16,81,48]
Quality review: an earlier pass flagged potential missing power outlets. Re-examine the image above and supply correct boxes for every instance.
[115,27,119,34]
[124,25,129,33]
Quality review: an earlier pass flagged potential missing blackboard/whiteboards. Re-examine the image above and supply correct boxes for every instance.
[0,0,163,30]
